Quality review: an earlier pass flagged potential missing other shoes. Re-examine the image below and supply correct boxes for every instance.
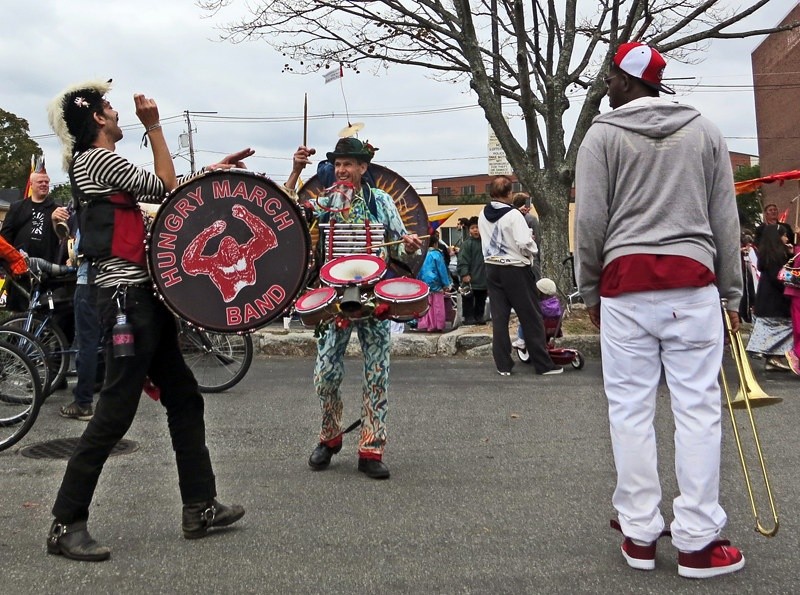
[511,338,526,350]
[496,369,510,376]
[767,355,791,370]
[541,365,563,376]
[784,350,800,376]
[27,377,68,391]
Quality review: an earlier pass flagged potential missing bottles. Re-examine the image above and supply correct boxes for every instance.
[112,314,136,360]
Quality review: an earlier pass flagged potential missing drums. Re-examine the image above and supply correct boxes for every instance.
[318,254,388,321]
[295,286,338,330]
[147,169,312,336]
[374,277,429,321]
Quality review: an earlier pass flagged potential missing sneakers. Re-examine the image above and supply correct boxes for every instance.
[678,540,745,578]
[610,519,672,570]
[59,401,94,420]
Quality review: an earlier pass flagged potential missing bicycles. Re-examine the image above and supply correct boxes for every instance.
[0,257,253,452]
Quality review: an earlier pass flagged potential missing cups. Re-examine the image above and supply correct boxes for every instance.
[284,317,291,330]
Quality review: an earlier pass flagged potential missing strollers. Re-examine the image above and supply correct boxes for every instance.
[517,291,585,369]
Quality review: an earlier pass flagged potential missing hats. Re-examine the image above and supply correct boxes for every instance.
[613,41,676,95]
[326,137,373,165]
[536,278,557,295]
[47,78,113,160]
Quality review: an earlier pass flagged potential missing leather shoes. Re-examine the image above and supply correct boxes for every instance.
[308,442,342,468]
[358,457,390,478]
[47,519,111,561]
[183,497,245,539]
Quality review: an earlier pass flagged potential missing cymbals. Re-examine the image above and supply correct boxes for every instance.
[338,122,364,137]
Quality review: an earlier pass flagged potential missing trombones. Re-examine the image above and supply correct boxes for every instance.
[720,297,783,538]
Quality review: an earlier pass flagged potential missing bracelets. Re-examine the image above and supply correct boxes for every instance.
[139,123,162,149]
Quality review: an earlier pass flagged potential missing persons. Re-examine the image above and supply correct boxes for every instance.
[0,138,800,482]
[45,79,245,561]
[574,43,746,579]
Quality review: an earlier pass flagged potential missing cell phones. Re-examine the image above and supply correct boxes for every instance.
[525,197,531,211]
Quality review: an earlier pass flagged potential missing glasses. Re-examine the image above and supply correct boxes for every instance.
[603,74,618,88]
